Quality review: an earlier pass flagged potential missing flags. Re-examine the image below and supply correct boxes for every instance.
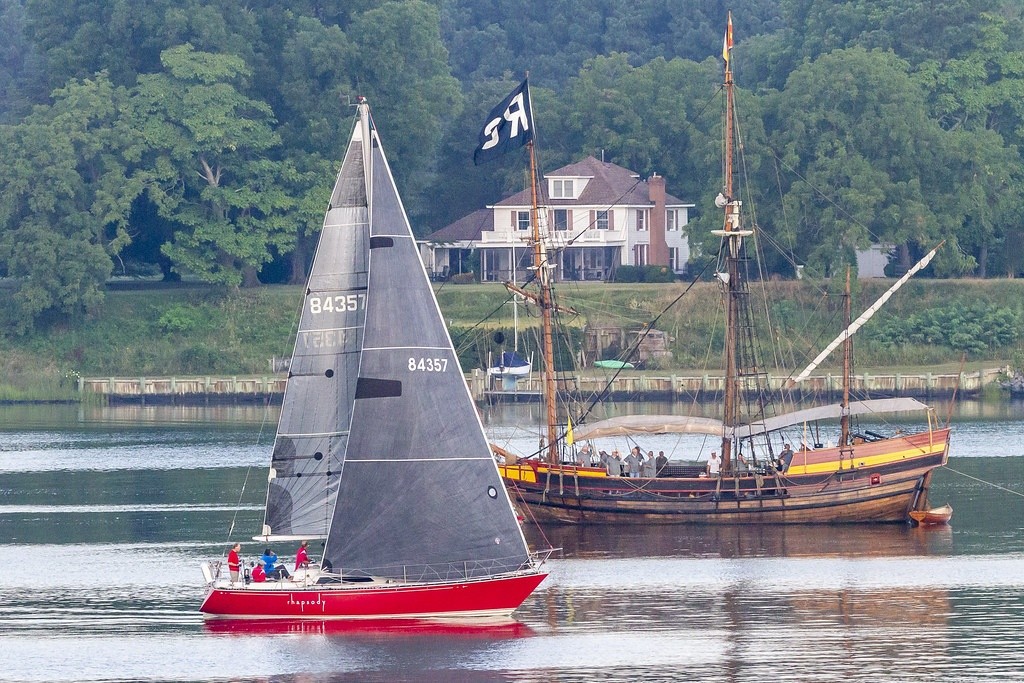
[473,78,533,166]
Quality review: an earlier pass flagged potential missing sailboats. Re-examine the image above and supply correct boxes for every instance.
[486,226,531,378]
[485,5,952,526]
[198,97,565,619]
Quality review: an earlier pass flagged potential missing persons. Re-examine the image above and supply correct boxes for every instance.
[228,541,311,582]
[577,445,669,478]
[737,442,795,473]
[707,450,722,478]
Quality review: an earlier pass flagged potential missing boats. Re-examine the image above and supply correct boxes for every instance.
[907,503,954,528]
